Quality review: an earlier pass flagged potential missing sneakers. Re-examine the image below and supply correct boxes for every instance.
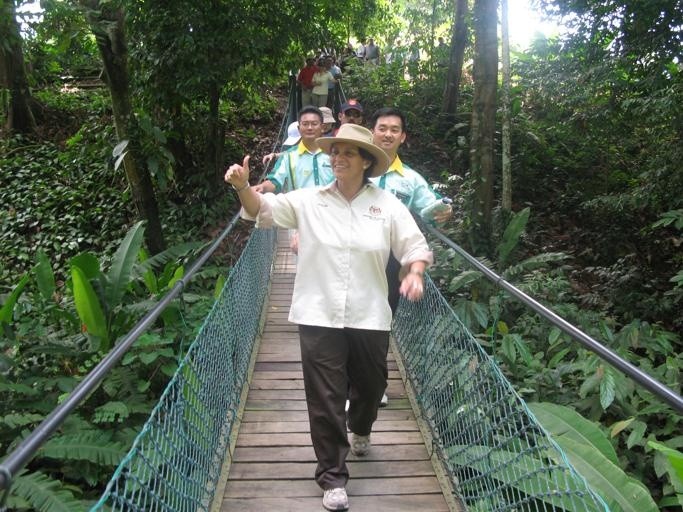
[353,430,370,456]
[379,393,389,407]
[323,487,349,510]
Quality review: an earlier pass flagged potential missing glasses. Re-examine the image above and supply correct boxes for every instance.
[345,109,360,117]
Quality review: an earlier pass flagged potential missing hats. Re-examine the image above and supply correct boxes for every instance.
[306,48,332,66]
[283,121,302,146]
[318,107,336,124]
[340,99,363,114]
[315,124,388,178]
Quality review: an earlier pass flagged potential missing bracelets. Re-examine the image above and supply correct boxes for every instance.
[408,268,425,277]
[229,180,250,192]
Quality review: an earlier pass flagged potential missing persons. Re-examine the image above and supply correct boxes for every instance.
[345,107,453,416]
[224,121,434,512]
[248,104,338,270]
[261,36,450,166]
